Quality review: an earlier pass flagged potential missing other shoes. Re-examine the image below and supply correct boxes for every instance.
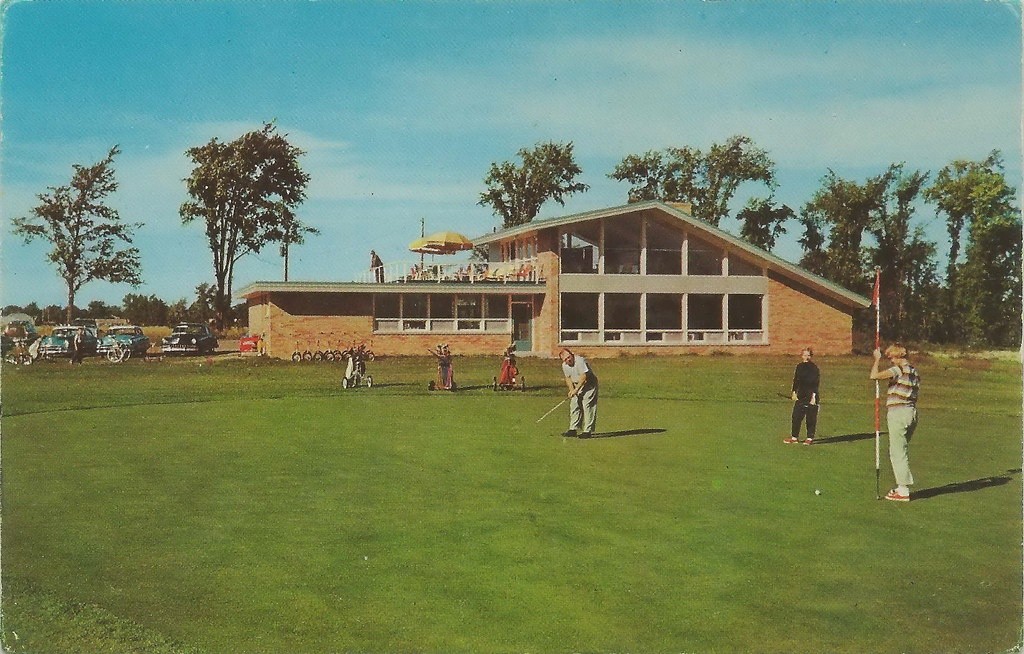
[578,432,592,439]
[561,430,578,438]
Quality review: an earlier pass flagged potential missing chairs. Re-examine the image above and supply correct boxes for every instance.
[411,264,535,281]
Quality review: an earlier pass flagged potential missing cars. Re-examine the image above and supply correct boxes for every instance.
[162,323,219,356]
[1,321,150,357]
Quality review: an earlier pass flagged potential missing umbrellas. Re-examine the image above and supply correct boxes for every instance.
[409,231,473,255]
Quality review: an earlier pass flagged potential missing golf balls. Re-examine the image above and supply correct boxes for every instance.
[815,489,821,495]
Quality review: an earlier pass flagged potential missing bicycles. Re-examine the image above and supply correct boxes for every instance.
[107,341,131,362]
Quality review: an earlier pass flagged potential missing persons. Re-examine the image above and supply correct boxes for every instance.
[369,250,384,283]
[559,349,598,439]
[69,328,85,364]
[783,347,820,446]
[870,344,920,501]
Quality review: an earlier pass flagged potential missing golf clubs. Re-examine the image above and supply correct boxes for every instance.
[776,392,821,412]
[534,397,568,424]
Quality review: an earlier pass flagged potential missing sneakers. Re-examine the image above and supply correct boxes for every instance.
[803,439,813,446]
[783,437,799,444]
[886,488,909,502]
